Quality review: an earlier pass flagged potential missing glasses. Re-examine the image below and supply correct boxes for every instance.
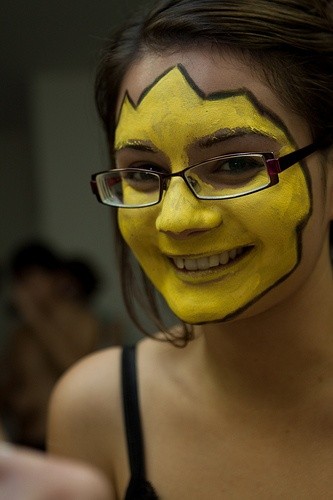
[90,137,321,208]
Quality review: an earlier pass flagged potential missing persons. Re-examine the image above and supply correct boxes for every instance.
[0,435,115,500]
[47,0,333,500]
[53,252,125,354]
[0,237,101,455]
